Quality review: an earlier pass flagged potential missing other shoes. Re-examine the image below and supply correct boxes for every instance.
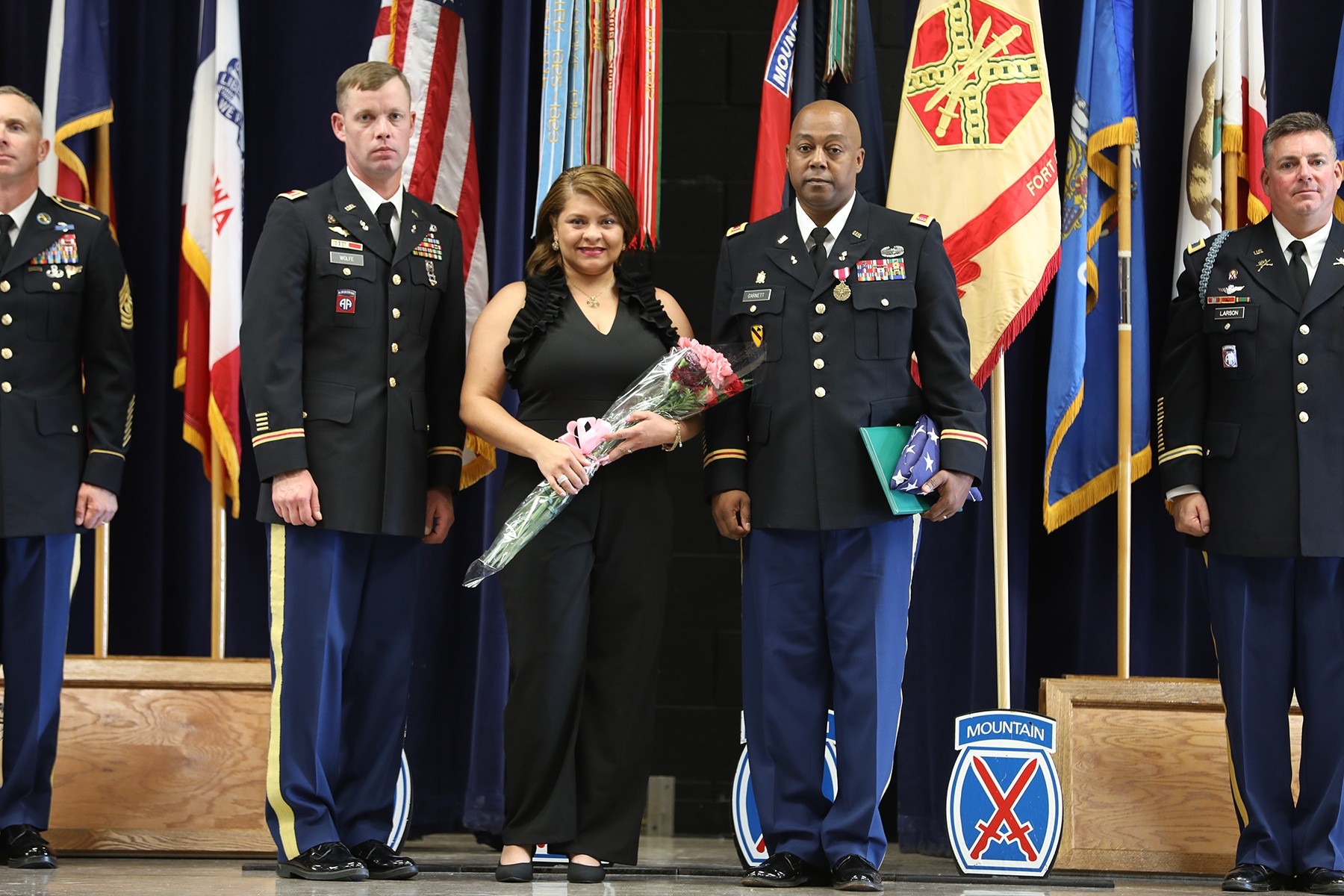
[494,843,537,883]
[565,851,605,882]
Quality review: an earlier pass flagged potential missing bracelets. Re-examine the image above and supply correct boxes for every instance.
[662,419,683,451]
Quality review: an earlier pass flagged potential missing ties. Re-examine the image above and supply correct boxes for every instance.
[375,202,397,257]
[1288,241,1311,302]
[808,228,830,277]
[0,214,15,270]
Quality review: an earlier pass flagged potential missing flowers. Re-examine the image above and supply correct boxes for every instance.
[459,338,754,586]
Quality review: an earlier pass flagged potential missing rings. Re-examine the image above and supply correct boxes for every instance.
[557,475,568,484]
[943,516,947,519]
[627,450,632,453]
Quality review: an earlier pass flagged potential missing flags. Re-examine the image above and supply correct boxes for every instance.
[885,1,1061,390]
[37,1,119,228]
[368,1,663,491]
[173,0,245,520]
[750,0,886,224]
[1042,0,1152,535]
[1326,20,1344,227]
[1155,112,1344,896]
[1172,0,1268,301]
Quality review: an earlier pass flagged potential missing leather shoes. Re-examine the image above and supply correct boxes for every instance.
[350,839,419,879]
[1221,863,1285,892]
[740,852,829,887]
[1284,867,1344,895]
[0,824,59,869]
[830,854,885,891]
[275,840,370,879]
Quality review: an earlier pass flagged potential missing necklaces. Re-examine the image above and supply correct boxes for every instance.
[567,275,615,308]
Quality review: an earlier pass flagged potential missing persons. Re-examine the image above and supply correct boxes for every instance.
[0,85,139,868]
[459,164,694,883]
[700,97,988,893]
[238,61,467,881]
